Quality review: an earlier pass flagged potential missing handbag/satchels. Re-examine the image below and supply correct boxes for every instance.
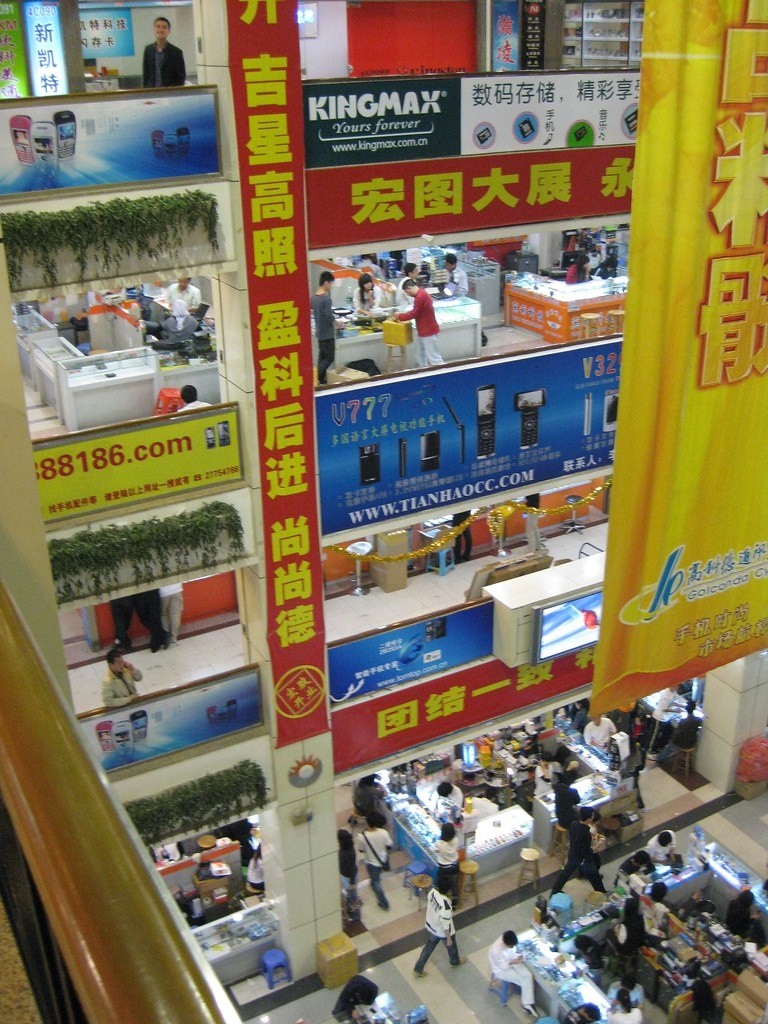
[382,860,390,872]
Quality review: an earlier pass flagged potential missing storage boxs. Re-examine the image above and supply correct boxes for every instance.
[734,781,768,801]
[724,991,765,1024]
[193,873,229,904]
[382,317,413,345]
[327,366,368,383]
[614,811,643,844]
[736,969,768,1007]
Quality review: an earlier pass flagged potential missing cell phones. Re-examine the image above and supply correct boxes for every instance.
[151,128,190,159]
[204,426,216,449]
[218,421,231,447]
[477,384,496,461]
[207,700,237,726]
[425,617,446,641]
[96,709,148,752]
[10,111,76,167]
[360,395,465,486]
[583,393,592,436]
[514,388,546,450]
[603,389,618,432]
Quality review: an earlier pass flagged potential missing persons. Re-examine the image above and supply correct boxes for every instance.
[555,682,703,811]
[443,255,487,347]
[107,582,183,655]
[220,818,265,891]
[413,875,466,978]
[177,385,213,411]
[555,781,582,830]
[526,493,541,551]
[164,277,201,313]
[395,262,418,306]
[453,510,472,563]
[430,822,461,910]
[395,279,445,367]
[595,256,619,280]
[310,270,344,387]
[487,929,539,1018]
[331,974,378,1019]
[430,782,465,825]
[565,255,591,284]
[335,774,394,918]
[533,751,553,797]
[548,804,768,1024]
[139,16,187,87]
[98,649,142,709]
[353,273,383,317]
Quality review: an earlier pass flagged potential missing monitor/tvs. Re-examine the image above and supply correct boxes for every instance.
[561,250,581,268]
[531,587,604,665]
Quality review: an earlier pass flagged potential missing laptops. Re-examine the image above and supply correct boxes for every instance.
[190,303,209,321]
[430,281,459,300]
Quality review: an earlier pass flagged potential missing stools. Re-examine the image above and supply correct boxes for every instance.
[346,543,371,595]
[410,874,433,911]
[549,822,569,867]
[535,1016,560,1024]
[349,810,365,829]
[578,313,600,339]
[260,948,291,989]
[197,834,216,850]
[489,971,520,1007]
[519,848,540,892]
[548,893,573,925]
[426,546,455,576]
[246,882,261,894]
[606,311,623,334]
[153,387,184,416]
[455,859,479,909]
[404,860,427,896]
[672,746,696,778]
[386,343,407,371]
[564,495,585,533]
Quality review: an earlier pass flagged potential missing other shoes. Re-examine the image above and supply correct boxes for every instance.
[649,754,657,761]
[413,970,427,977]
[377,901,389,909]
[452,956,466,969]
[522,1003,538,1016]
